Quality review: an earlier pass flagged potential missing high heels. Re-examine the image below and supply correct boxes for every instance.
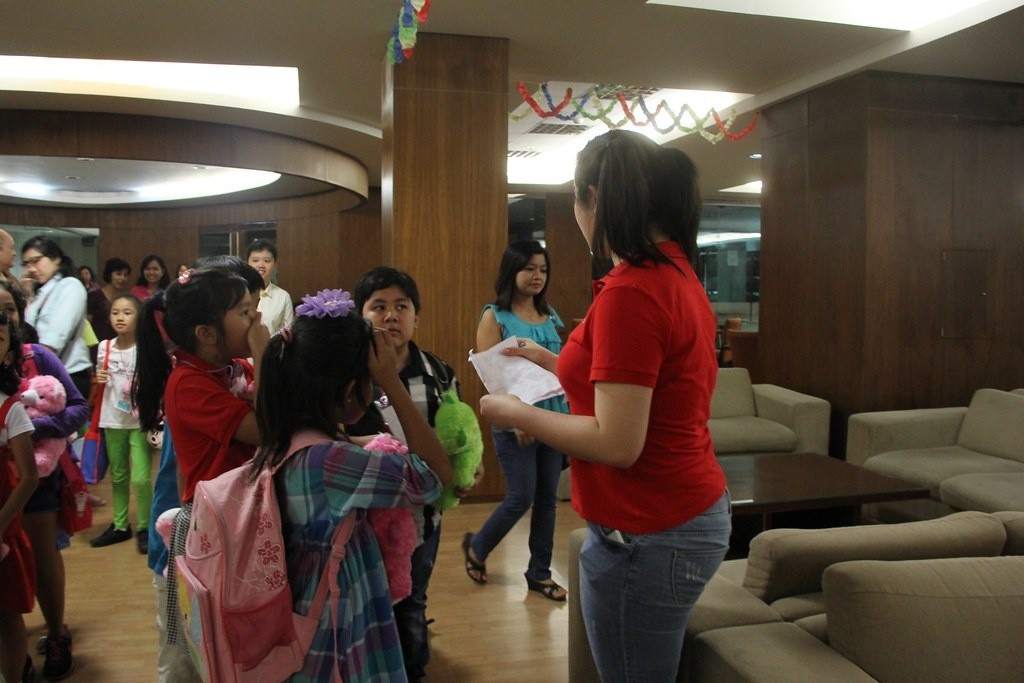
[461,532,490,586]
[523,573,567,601]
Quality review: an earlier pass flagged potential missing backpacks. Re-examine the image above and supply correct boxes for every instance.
[172,426,356,683]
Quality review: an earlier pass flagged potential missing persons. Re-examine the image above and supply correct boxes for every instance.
[478,128,732,683]
[0,227,460,683]
[461,240,570,601]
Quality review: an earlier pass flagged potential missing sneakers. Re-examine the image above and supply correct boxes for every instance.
[36,622,49,655]
[89,522,133,548]
[42,623,74,681]
[135,529,148,555]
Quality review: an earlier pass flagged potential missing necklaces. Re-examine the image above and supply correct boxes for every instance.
[514,304,536,319]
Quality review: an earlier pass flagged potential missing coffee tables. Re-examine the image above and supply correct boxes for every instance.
[714,452,932,563]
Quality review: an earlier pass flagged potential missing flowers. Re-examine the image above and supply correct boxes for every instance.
[295,288,356,318]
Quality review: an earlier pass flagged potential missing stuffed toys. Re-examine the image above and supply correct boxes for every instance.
[20,375,67,475]
[146,421,164,449]
[363,431,425,606]
[434,389,484,509]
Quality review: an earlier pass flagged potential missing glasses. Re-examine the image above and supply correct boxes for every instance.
[20,255,45,267]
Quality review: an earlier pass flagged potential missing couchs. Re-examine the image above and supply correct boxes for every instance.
[676,367,1024,683]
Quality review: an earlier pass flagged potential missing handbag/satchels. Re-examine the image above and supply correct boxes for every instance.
[84,317,100,348]
[58,451,93,534]
[80,428,109,486]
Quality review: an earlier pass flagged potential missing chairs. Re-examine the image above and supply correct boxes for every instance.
[716,317,743,368]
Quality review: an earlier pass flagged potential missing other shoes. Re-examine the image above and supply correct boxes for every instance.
[23,653,36,681]
[90,493,108,508]
